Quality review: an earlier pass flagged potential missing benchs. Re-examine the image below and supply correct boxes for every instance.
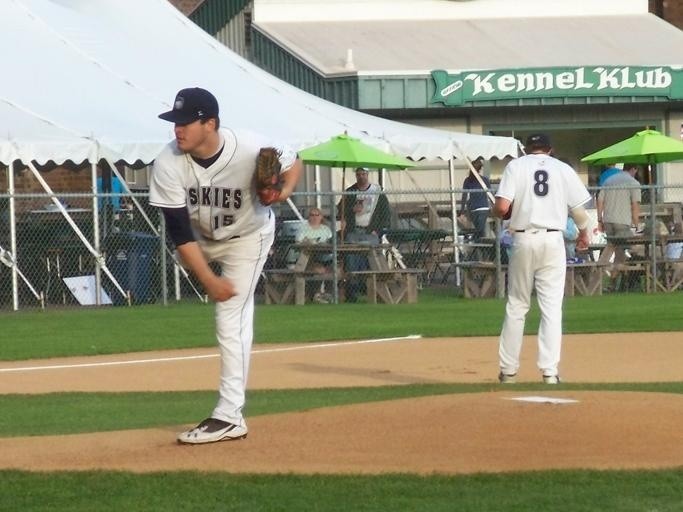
[563,207,682,298]
[257,204,510,306]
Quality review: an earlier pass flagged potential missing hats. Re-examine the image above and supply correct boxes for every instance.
[353,167,369,172]
[155,86,220,131]
[524,134,551,147]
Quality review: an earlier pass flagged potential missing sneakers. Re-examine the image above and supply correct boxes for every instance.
[496,371,516,384]
[542,373,562,384]
[173,414,250,447]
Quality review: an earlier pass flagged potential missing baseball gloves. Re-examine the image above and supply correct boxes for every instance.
[255,147,285,206]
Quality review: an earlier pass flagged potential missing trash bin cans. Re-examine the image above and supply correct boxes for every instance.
[101,233,160,306]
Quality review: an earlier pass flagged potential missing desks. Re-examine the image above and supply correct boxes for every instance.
[606,236,681,263]
[283,243,398,305]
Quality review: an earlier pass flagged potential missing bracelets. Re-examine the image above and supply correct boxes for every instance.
[598,218,602,222]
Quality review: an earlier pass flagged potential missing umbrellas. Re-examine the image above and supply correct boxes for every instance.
[297,130,417,294]
[581,124,683,294]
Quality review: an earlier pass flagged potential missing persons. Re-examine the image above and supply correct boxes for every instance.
[597,164,643,294]
[490,133,592,384]
[148,88,303,445]
[295,208,342,304]
[96,173,127,232]
[598,164,622,187]
[460,160,493,243]
[336,168,391,304]
[566,217,578,261]
